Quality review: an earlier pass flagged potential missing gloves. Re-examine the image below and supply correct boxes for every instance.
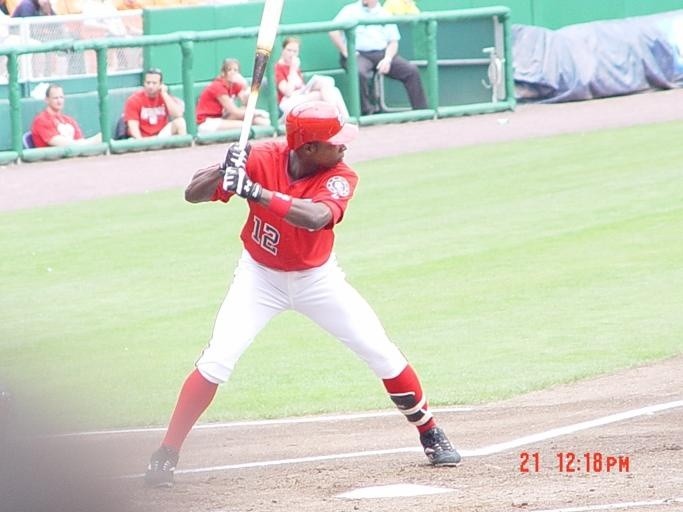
[221,166,262,202]
[220,142,250,171]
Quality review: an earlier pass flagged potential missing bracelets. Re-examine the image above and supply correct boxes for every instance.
[265,191,291,217]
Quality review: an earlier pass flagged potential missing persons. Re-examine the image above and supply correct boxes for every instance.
[193,57,271,132]
[120,66,187,140]
[324,0,429,116]
[381,1,421,16]
[0,0,48,84]
[114,0,146,35]
[80,0,143,73]
[271,35,349,119]
[142,97,464,490]
[30,81,103,150]
[10,1,87,79]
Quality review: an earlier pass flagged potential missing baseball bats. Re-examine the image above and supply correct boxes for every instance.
[228,0,284,194]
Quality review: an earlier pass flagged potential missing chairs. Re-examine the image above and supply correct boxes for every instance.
[23,130,35,148]
[116,116,128,138]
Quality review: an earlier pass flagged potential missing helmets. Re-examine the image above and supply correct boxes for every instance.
[283,101,360,150]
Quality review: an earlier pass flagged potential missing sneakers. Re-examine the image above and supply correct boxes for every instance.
[419,427,461,466]
[144,443,179,488]
